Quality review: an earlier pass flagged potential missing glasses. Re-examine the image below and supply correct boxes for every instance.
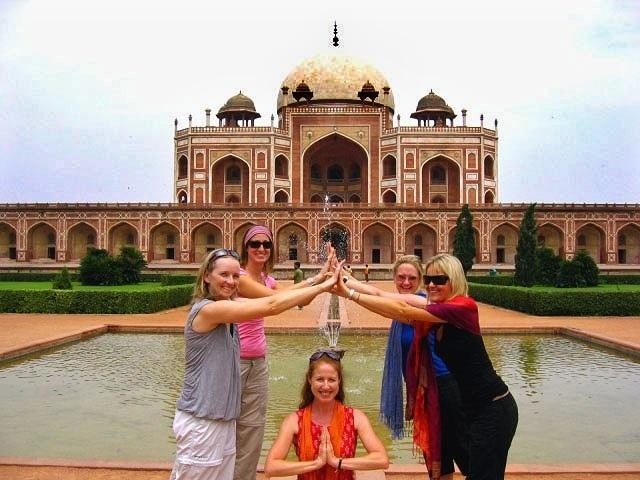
[208,248,240,264]
[422,274,449,286]
[247,240,271,249]
[309,350,342,363]
